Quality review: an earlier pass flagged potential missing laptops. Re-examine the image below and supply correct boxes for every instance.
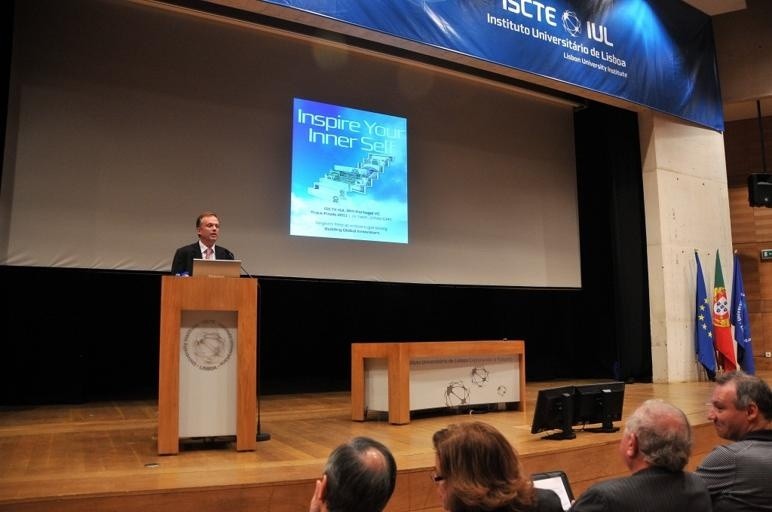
[192,258,241,278]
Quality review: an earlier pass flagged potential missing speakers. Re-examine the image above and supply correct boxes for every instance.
[746,173,772,207]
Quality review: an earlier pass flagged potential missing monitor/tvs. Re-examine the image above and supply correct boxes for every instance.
[532,385,580,440]
[528,471,575,511]
[576,383,625,433]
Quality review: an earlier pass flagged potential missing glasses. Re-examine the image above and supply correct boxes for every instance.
[430,474,447,485]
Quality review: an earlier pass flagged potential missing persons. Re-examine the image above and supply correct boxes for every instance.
[428,421,566,511]
[170,211,236,275]
[567,398,714,511]
[307,434,398,511]
[695,368,772,511]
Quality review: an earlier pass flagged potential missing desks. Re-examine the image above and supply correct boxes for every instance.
[350,340,526,425]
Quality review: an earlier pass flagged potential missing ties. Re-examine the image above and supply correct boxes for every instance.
[206,249,213,260]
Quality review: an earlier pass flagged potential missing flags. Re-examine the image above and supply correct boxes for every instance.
[692,249,717,375]
[730,254,755,376]
[710,251,738,375]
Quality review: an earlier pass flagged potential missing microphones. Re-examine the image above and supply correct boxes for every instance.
[224,249,252,278]
